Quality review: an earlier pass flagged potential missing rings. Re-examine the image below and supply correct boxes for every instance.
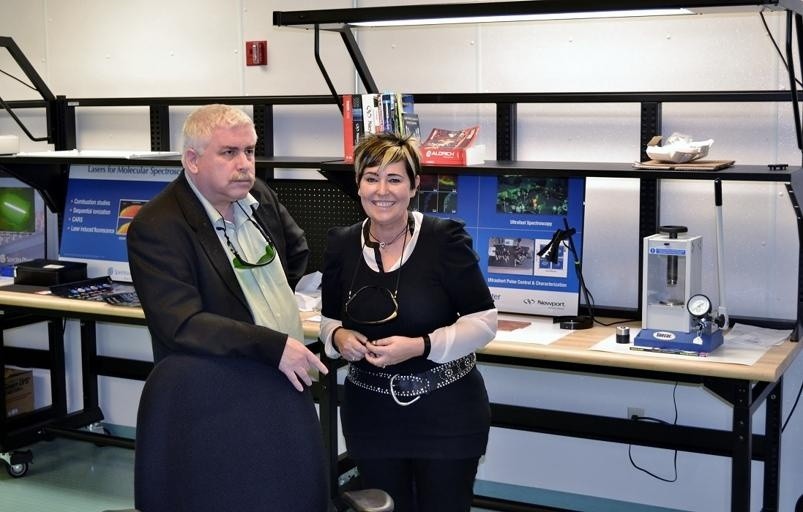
[379,362,387,369]
[350,355,355,361]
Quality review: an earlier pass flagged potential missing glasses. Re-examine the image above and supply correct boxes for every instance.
[226,216,275,269]
[345,284,397,325]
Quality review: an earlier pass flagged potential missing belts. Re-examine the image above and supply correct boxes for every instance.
[347,354,477,407]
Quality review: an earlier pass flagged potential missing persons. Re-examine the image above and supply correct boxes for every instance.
[319,133,500,511]
[127,104,328,394]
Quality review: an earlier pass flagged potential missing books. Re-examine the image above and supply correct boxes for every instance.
[633,156,735,174]
[341,93,485,168]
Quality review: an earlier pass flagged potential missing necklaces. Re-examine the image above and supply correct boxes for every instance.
[367,229,406,248]
